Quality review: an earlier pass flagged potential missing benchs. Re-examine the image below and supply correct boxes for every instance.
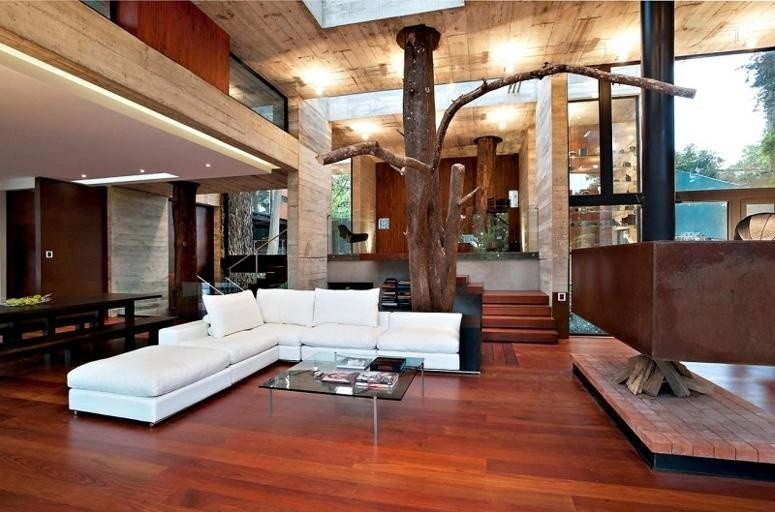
[0,311,183,365]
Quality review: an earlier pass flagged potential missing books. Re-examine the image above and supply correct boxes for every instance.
[370,356,407,373]
[322,371,360,385]
[356,370,398,387]
[380,277,411,305]
[405,357,425,369]
[336,356,372,370]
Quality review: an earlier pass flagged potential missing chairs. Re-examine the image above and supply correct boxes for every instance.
[337,224,368,253]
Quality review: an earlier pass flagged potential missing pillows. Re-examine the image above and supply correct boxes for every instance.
[201,287,381,339]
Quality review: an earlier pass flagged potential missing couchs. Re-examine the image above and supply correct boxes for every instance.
[64,309,462,428]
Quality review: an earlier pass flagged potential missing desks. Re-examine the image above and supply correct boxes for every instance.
[0,292,162,351]
